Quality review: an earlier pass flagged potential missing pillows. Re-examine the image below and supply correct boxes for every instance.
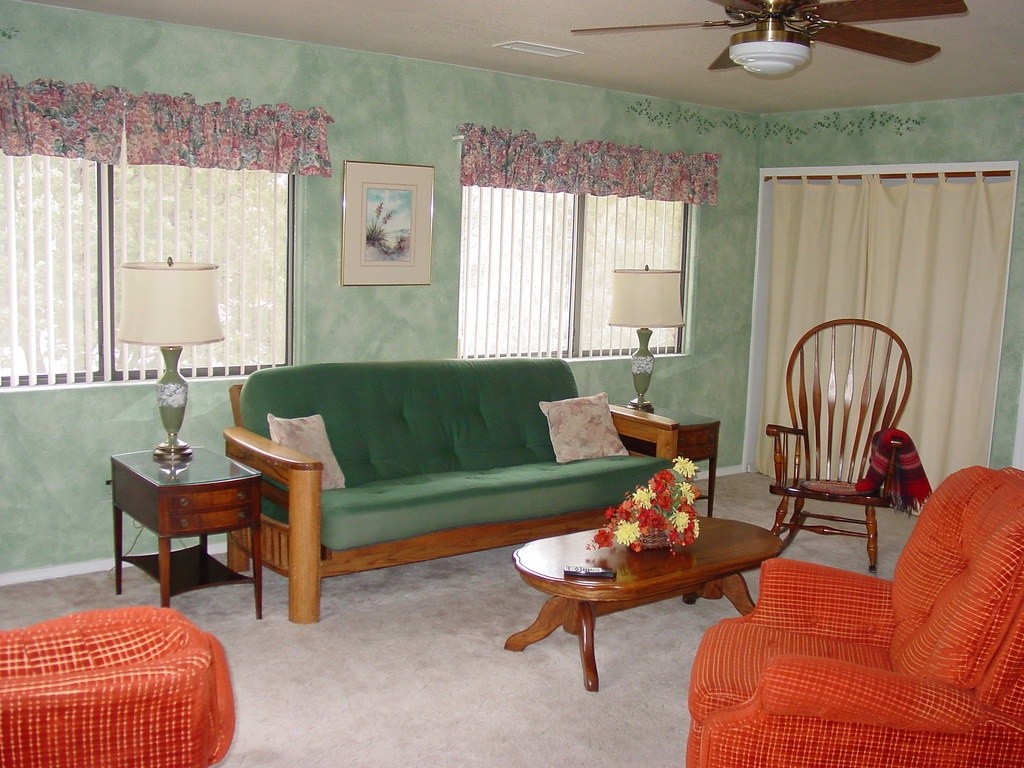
[539,391,630,465]
[267,412,346,491]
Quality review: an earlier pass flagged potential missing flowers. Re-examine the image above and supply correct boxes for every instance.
[585,455,703,556]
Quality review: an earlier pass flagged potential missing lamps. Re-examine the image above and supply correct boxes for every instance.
[606,264,686,414]
[729,23,812,76]
[117,256,225,460]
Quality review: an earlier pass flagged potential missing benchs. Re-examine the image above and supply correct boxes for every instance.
[223,358,679,624]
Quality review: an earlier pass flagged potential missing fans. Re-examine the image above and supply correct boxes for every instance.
[570,0,968,70]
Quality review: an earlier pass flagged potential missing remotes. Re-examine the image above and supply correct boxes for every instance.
[564,566,618,578]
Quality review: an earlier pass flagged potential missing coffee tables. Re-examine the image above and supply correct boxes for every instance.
[503,515,784,692]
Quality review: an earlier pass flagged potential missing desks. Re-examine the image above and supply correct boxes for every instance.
[618,407,722,518]
[110,446,263,620]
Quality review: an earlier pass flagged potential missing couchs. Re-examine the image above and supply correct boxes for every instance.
[686,465,1024,768]
[0,606,236,768]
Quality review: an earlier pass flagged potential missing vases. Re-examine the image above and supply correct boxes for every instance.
[625,527,670,550]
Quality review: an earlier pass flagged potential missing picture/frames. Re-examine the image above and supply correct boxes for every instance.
[339,160,435,287]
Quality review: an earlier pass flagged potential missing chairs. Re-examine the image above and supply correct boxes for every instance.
[766,318,932,574]
